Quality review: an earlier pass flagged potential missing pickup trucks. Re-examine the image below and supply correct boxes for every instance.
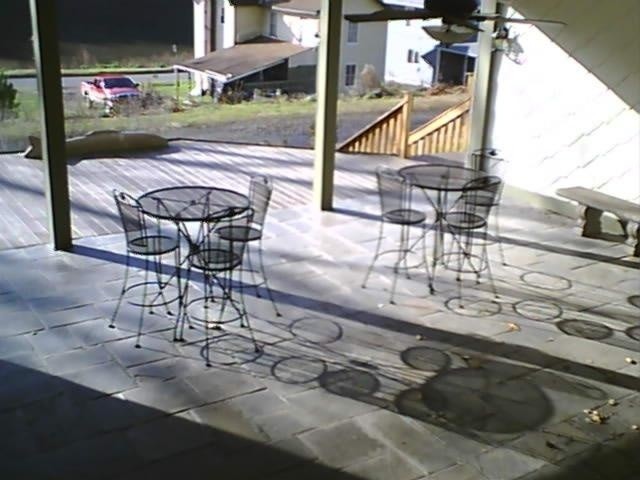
[78,73,144,116]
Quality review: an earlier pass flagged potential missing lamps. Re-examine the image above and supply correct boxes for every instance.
[422,21,477,49]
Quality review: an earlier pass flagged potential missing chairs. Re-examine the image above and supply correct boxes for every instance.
[108,172,282,368]
[361,147,510,310]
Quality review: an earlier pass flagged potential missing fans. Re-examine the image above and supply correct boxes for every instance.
[343,0,568,33]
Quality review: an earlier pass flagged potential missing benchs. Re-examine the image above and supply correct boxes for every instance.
[556,184,640,257]
